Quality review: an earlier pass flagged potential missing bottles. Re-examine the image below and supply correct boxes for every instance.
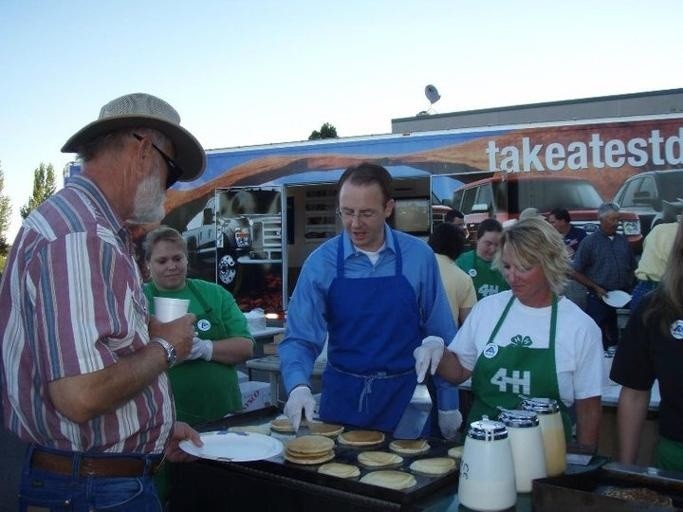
[495,405,547,493]
[517,393,567,477]
[458,414,518,512]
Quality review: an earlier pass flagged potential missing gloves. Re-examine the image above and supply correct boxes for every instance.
[414,335,445,385]
[281,387,317,433]
[188,336,215,363]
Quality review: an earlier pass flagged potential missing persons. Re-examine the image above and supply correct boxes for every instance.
[609,216,683,475]
[548,206,587,264]
[445,208,467,229]
[0,92,205,512]
[629,213,681,317]
[413,217,604,456]
[277,162,464,440]
[427,223,477,332]
[567,202,638,351]
[456,219,514,304]
[142,224,256,510]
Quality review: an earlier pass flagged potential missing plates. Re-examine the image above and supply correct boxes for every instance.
[602,290,631,308]
[178,430,284,461]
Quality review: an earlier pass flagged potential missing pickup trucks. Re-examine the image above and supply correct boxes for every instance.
[181,196,283,289]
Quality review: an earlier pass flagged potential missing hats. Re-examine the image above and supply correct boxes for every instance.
[59,92,207,184]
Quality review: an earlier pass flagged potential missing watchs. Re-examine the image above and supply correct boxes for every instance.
[147,336,180,370]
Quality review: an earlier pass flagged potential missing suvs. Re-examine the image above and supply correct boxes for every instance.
[613,169,683,235]
[431,189,454,229]
[452,175,643,251]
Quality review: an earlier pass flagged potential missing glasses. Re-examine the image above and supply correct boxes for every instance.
[333,202,386,220]
[132,129,181,190]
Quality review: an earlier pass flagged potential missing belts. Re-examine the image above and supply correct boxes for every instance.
[23,449,170,481]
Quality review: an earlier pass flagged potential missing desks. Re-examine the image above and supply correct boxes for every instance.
[185,405,682,512]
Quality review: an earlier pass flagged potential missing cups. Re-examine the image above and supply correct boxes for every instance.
[154,297,190,322]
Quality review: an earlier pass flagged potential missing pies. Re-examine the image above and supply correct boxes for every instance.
[359,469,417,490]
[337,430,385,446]
[448,444,464,457]
[389,440,431,453]
[318,462,361,479]
[358,450,403,466]
[283,435,335,465]
[311,423,344,437]
[409,458,456,475]
[271,419,293,432]
